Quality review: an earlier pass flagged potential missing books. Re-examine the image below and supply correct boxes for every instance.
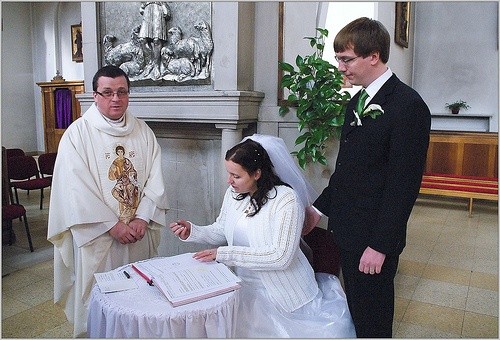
[132,253,242,306]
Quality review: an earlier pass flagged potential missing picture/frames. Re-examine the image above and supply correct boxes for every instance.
[71,24,84,61]
[395,2,411,48]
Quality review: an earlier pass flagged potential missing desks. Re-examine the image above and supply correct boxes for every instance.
[88,258,240,339]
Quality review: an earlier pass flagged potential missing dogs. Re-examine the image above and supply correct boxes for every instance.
[101,18,213,81]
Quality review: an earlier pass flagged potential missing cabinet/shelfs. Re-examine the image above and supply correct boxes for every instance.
[36,81,86,153]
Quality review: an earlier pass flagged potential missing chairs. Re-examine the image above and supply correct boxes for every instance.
[2,181,34,253]
[38,153,58,197]
[7,156,50,221]
[302,226,340,277]
[5,149,31,196]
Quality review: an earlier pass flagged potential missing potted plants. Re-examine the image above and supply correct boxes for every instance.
[445,99,471,114]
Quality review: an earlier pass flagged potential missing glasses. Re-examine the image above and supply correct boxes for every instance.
[97,90,130,98]
[335,49,374,66]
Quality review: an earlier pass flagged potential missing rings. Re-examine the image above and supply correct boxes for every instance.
[210,252,212,255]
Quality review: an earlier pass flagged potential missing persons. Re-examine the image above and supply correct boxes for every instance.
[139,1,172,79]
[300,15,432,339]
[48,65,167,338]
[169,133,357,339]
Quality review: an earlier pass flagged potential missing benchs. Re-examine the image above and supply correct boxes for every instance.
[419,172,499,218]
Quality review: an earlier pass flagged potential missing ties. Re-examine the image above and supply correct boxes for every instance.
[356,91,369,115]
